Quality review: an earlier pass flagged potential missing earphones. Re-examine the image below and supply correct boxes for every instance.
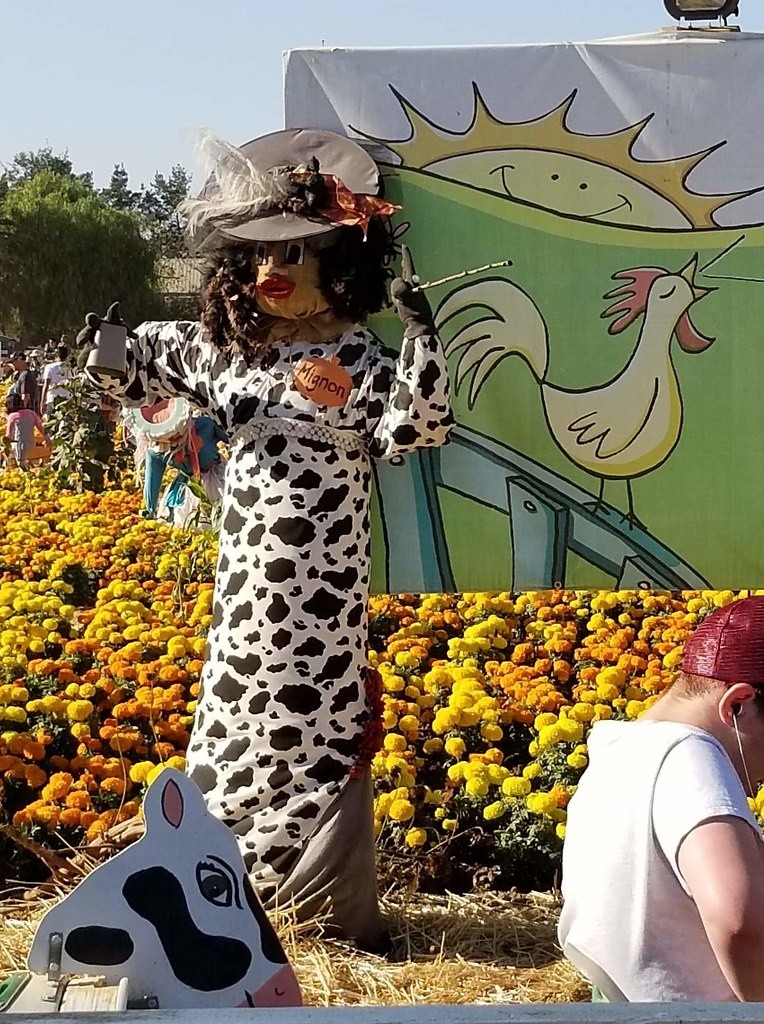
[731,703,743,718]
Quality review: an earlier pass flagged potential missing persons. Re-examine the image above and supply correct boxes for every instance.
[0,333,229,520]
[557,597,764,1007]
[76,127,457,957]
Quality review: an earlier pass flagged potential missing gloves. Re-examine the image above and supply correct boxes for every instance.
[391,243,439,339]
[76,301,139,349]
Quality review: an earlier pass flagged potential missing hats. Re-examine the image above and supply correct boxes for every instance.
[58,347,68,357]
[682,596,764,686]
[7,352,26,363]
